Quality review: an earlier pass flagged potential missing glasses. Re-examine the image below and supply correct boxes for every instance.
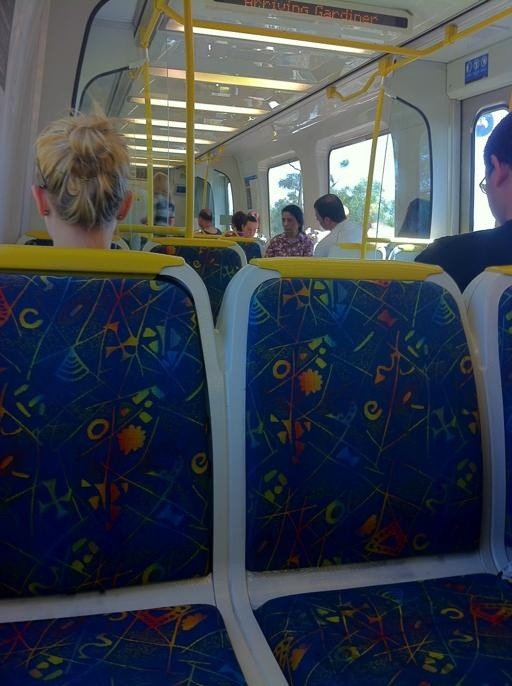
[479,175,489,194]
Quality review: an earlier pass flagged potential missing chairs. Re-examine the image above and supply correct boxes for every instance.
[0,230,512,686]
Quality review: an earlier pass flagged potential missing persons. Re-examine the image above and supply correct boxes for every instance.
[29,111,133,250]
[412,109,512,294]
[137,171,384,260]
